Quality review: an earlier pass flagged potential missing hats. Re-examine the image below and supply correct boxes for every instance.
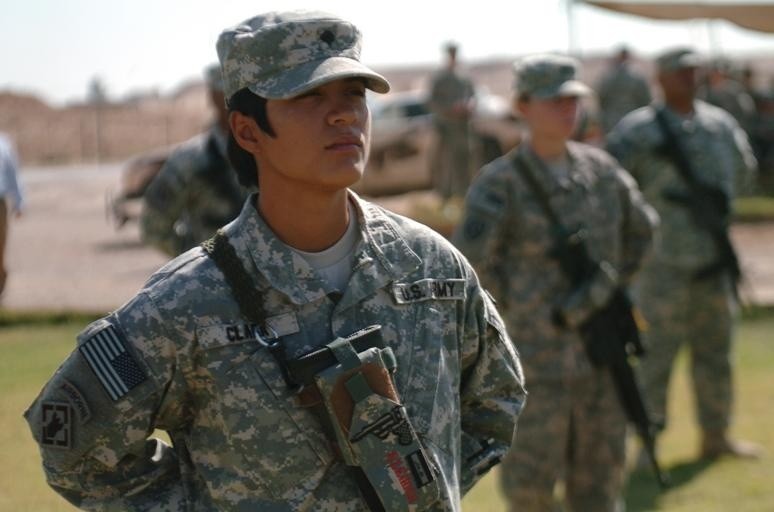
[204,9,391,110]
[514,54,595,100]
[657,46,705,71]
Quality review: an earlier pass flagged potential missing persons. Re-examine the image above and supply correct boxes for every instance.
[22,11,529,512]
[499,45,772,196]
[602,45,763,478]
[0,136,23,293]
[139,66,260,259]
[427,43,480,210]
[451,52,661,512]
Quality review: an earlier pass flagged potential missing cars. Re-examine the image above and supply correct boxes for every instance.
[106,85,526,229]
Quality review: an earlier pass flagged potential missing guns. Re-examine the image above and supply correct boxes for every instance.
[288,324,444,512]
[655,137,752,315]
[549,229,669,490]
